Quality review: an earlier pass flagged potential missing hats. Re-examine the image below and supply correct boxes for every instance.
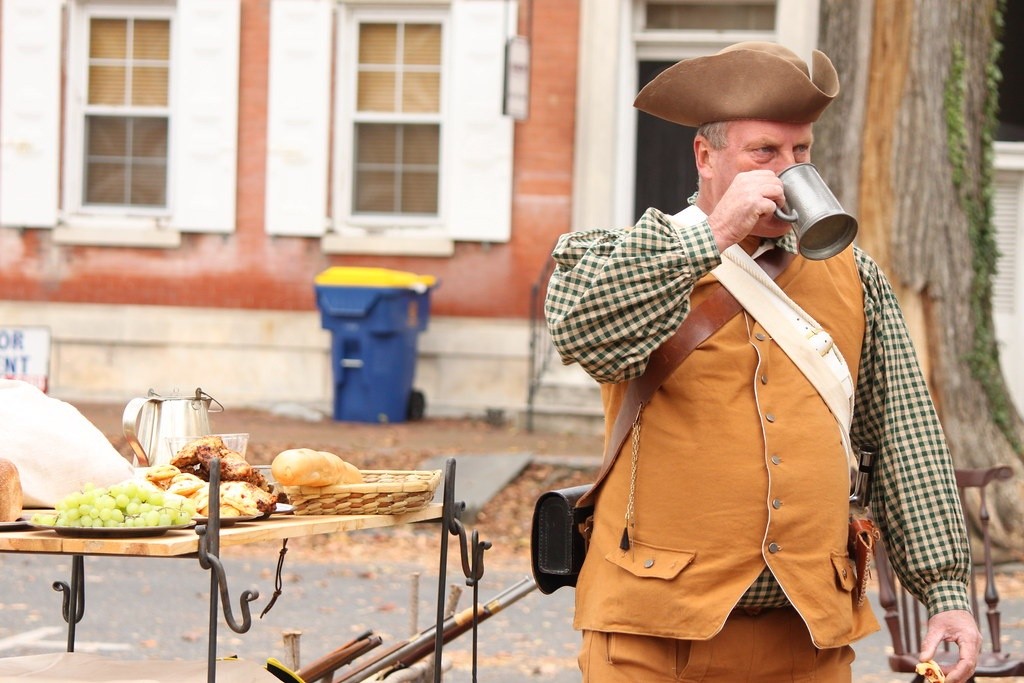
[634,41,843,129]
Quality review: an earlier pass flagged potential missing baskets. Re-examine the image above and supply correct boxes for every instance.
[286,469,446,515]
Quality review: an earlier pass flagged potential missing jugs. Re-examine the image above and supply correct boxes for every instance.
[120,385,224,469]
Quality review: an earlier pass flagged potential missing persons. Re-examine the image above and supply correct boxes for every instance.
[541,38,986,682]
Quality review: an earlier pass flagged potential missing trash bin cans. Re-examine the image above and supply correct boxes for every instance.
[313,265,442,423]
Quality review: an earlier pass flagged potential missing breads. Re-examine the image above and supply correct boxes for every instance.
[272,448,363,485]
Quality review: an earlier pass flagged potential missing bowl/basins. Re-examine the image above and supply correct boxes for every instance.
[163,431,251,464]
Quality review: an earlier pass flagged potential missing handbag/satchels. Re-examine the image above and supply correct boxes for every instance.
[529,481,595,594]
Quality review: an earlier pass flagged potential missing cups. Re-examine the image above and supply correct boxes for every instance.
[771,162,856,262]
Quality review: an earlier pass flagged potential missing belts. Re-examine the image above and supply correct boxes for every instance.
[727,607,794,622]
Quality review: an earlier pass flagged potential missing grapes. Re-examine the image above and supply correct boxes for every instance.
[29,483,196,528]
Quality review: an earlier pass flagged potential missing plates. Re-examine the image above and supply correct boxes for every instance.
[192,511,265,525]
[26,517,196,538]
[0,511,34,529]
[258,501,297,519]
